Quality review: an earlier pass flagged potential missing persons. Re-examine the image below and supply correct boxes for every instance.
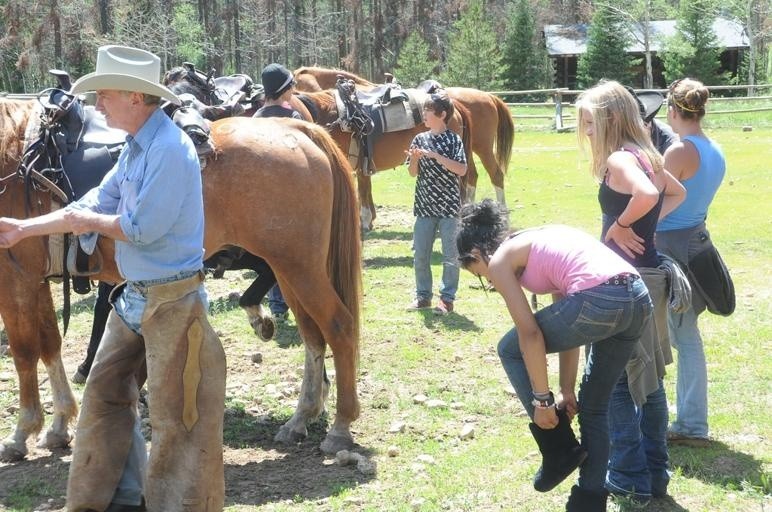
[456,198,654,512]
[574,80,687,502]
[655,77,725,445]
[626,87,677,155]
[252,63,303,324]
[404,92,468,315]
[0,45,226,512]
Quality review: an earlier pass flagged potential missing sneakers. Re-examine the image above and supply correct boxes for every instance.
[85,495,145,511]
[271,313,287,321]
[405,297,431,312]
[432,300,453,314]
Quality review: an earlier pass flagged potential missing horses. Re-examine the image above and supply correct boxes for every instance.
[238,91,473,236]
[70,250,278,386]
[292,66,515,232]
[0,98,364,463]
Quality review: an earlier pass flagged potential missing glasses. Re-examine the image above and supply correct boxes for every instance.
[670,78,697,92]
[666,431,708,448]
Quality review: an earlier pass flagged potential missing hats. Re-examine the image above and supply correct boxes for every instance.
[626,87,664,124]
[261,63,293,95]
[431,94,452,114]
[71,46,182,106]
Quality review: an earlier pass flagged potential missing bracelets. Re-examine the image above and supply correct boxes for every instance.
[616,217,629,229]
[530,390,557,409]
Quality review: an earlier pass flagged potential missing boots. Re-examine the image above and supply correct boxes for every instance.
[565,485,609,512]
[529,410,588,492]
